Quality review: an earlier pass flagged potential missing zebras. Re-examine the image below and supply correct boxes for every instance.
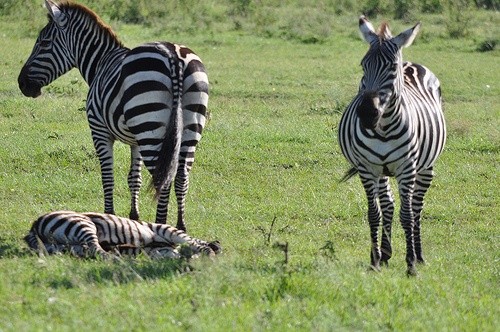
[23,209,220,282]
[339,13,446,279]
[17,0,209,233]
